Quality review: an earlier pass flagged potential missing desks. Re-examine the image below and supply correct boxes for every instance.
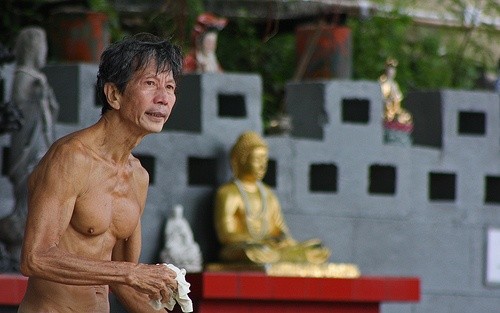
[171,271,422,313]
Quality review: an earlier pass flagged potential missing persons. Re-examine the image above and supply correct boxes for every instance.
[161,202,203,271]
[211,133,331,265]
[18,33,197,312]
[374,58,414,144]
[179,10,227,73]
[0,25,60,275]
